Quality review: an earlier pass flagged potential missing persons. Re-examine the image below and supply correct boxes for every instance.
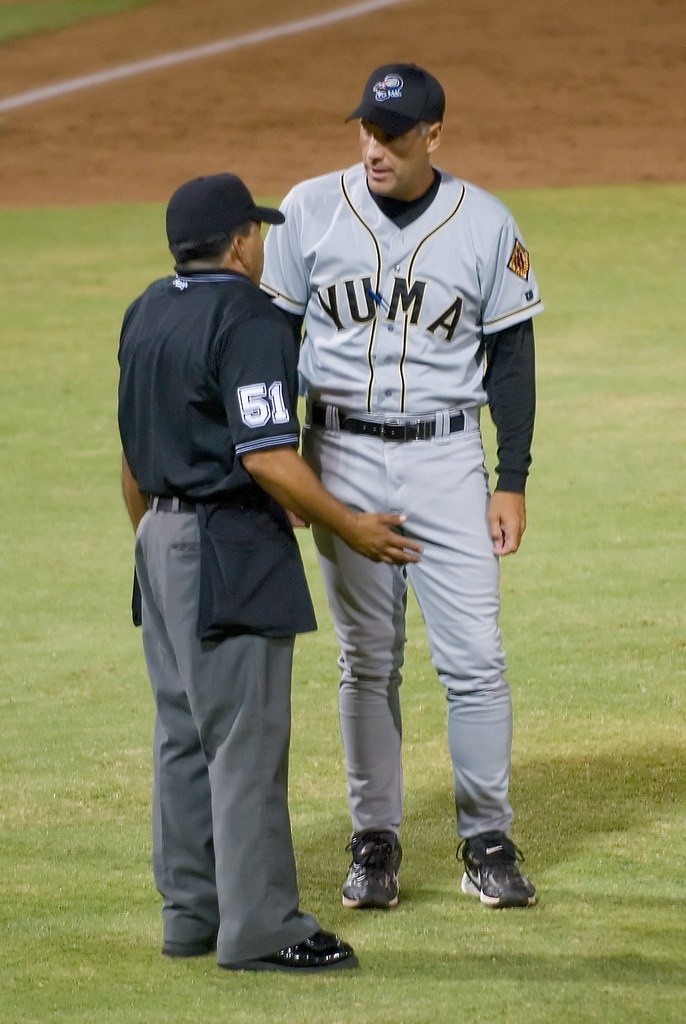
[117,169,424,972]
[250,63,544,905]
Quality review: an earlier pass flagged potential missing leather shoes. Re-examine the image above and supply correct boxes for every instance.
[221,930,359,975]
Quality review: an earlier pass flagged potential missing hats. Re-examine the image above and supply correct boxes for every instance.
[166,174,286,243]
[345,63,446,125]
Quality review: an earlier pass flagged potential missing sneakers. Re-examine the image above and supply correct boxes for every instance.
[455,830,536,906]
[341,829,402,908]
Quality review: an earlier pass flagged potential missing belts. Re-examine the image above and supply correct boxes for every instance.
[146,494,197,513]
[304,403,464,443]
